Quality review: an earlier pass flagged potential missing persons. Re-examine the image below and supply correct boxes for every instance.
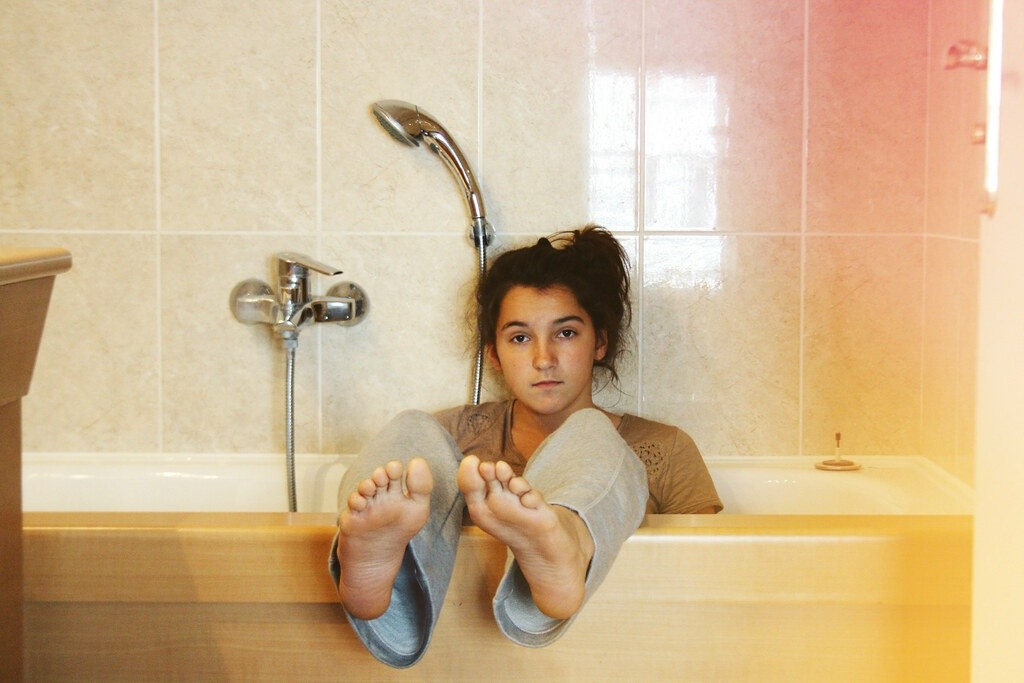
[328,225,722,667]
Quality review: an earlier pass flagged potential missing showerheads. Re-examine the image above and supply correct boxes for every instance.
[371,98,486,220]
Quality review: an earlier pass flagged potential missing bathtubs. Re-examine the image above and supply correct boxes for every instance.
[21,451,971,683]
